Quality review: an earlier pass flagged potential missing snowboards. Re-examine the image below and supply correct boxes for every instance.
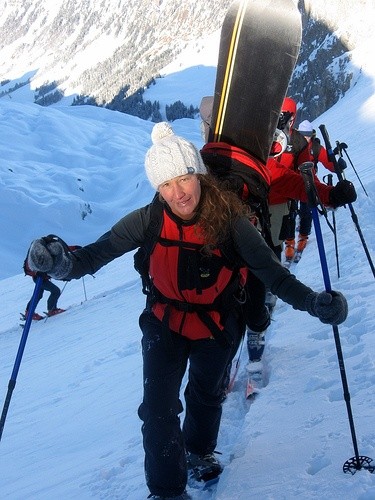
[206,0,303,167]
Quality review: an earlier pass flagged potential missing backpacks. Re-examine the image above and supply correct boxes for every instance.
[134,140,275,333]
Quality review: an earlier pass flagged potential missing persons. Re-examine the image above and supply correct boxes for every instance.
[264,96,348,312]
[285,119,347,258]
[198,95,357,388]
[28,122,348,500]
[20,253,66,319]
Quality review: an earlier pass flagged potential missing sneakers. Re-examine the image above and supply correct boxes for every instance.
[184,451,224,483]
[284,238,295,258]
[296,233,309,251]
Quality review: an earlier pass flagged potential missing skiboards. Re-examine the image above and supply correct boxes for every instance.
[226,338,264,400]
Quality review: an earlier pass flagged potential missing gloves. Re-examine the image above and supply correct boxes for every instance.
[27,237,74,281]
[337,157,348,174]
[304,290,349,326]
[328,178,358,209]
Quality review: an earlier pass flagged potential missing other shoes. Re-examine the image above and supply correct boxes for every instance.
[47,308,64,316]
[245,358,264,394]
[25,309,42,320]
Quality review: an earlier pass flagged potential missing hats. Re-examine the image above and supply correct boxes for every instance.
[144,121,209,192]
[296,119,314,137]
